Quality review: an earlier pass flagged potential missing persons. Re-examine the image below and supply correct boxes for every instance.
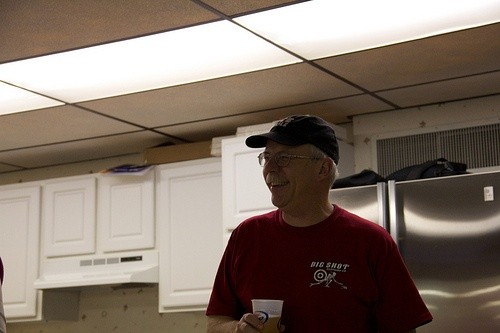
[206,115,432,333]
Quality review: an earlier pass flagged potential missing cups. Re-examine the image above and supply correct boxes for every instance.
[252,300,283,333]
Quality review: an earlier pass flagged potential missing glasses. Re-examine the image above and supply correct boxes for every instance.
[258,152,324,167]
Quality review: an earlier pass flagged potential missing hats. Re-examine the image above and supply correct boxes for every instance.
[245,115,339,166]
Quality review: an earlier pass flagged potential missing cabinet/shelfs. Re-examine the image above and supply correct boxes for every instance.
[0,120,354,323]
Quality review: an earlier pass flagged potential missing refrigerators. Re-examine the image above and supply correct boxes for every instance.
[328,171,500,333]
